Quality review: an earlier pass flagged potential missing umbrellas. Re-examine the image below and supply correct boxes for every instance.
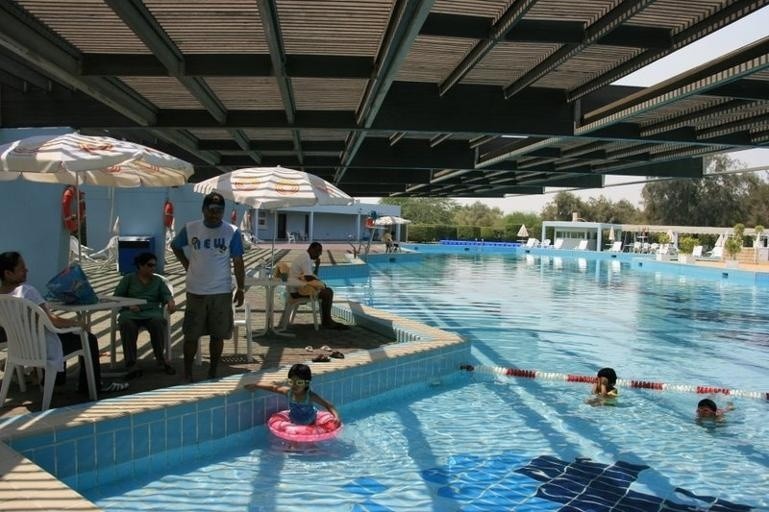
[0,131,197,275]
[192,162,356,278]
[373,215,412,233]
[516,223,529,240]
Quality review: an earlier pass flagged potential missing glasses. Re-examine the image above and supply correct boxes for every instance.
[144,262,157,269]
[698,407,717,415]
[288,377,310,387]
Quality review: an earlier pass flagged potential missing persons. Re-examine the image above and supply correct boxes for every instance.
[583,367,618,407]
[169,192,248,384]
[286,241,344,330]
[244,361,343,449]
[391,231,401,254]
[111,251,177,376]
[382,229,393,254]
[0,251,107,398]
[694,398,735,430]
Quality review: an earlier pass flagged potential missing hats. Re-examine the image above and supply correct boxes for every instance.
[204,191,227,212]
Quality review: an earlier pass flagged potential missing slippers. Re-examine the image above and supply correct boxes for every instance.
[303,344,345,363]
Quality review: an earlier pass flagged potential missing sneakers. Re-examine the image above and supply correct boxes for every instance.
[101,382,131,394]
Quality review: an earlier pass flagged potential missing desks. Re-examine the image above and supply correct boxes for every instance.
[243,278,296,339]
[517,240,526,244]
[47,294,147,386]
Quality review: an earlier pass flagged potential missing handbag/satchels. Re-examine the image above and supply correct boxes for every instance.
[46,262,99,305]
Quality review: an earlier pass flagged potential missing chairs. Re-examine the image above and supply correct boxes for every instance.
[381,233,401,255]
[523,238,723,262]
[286,232,296,244]
[110,272,174,369]
[195,288,253,367]
[0,294,98,412]
[273,263,319,331]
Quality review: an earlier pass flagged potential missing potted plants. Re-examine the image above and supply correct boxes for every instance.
[725,236,743,268]
[752,225,764,248]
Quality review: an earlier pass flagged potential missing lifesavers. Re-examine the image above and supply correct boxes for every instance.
[63,186,85,229]
[164,202,173,227]
[231,209,236,222]
[268,409,344,442]
